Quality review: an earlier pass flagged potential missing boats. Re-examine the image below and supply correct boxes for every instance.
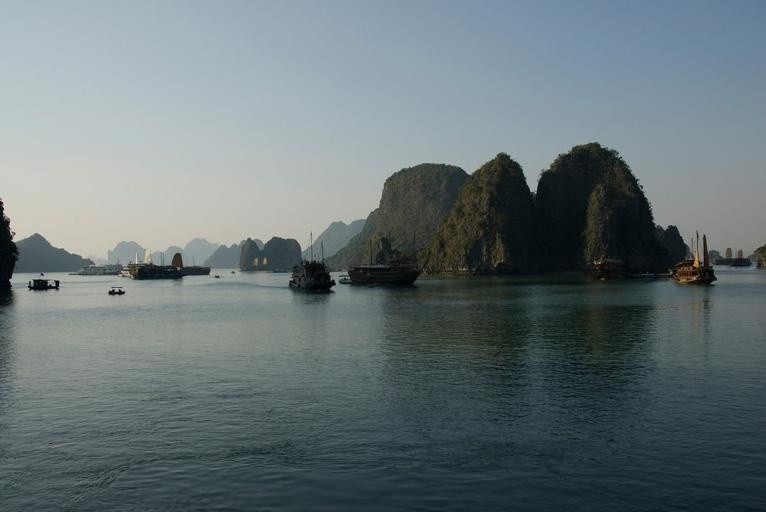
[287,228,337,291]
[118,248,210,275]
[26,272,59,290]
[346,231,432,284]
[711,256,754,266]
[126,249,184,279]
[107,286,126,295]
[67,249,122,276]
[339,277,351,285]
[590,254,625,280]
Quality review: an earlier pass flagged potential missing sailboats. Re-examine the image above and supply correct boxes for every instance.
[668,231,717,285]
